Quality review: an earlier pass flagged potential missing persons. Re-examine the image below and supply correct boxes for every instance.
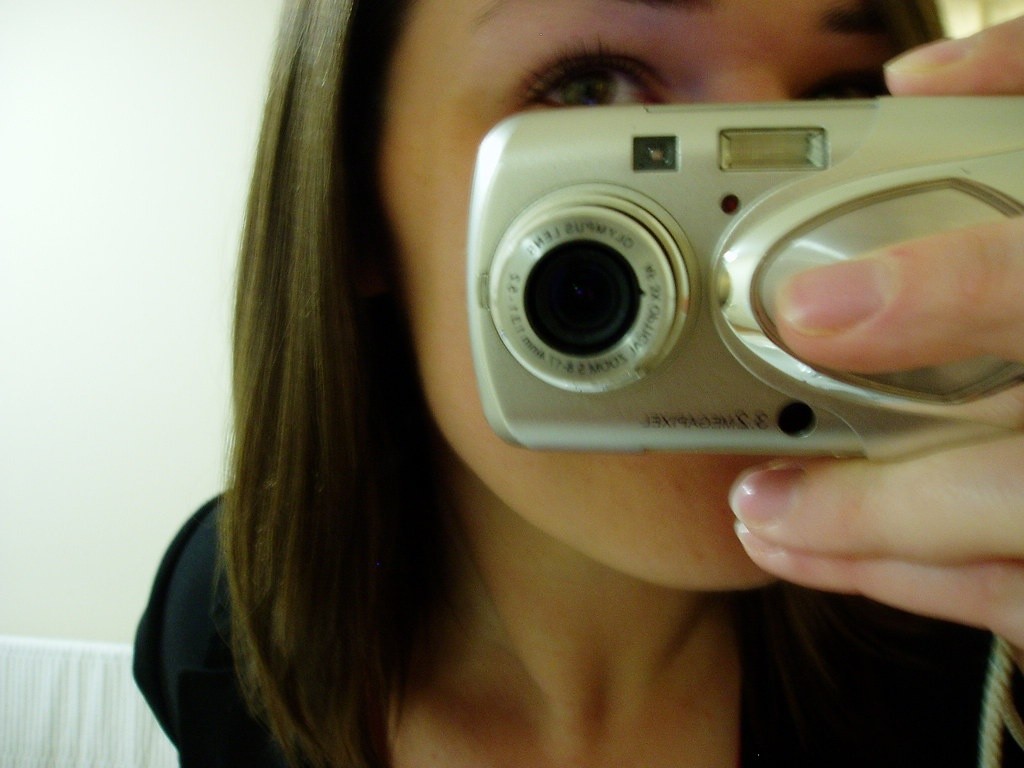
[132,0,1024,767]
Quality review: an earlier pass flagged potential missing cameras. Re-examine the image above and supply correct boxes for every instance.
[467,95,1024,456]
